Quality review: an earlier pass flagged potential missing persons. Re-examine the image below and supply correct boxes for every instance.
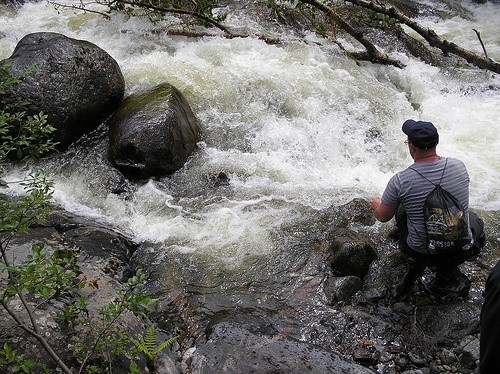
[479,258,499,374]
[371,119,486,267]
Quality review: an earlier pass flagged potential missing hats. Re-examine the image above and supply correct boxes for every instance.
[402,119,439,147]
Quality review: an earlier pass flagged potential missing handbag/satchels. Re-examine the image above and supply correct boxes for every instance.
[465,211,487,261]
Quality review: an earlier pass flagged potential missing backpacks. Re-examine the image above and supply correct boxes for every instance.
[402,157,472,257]
